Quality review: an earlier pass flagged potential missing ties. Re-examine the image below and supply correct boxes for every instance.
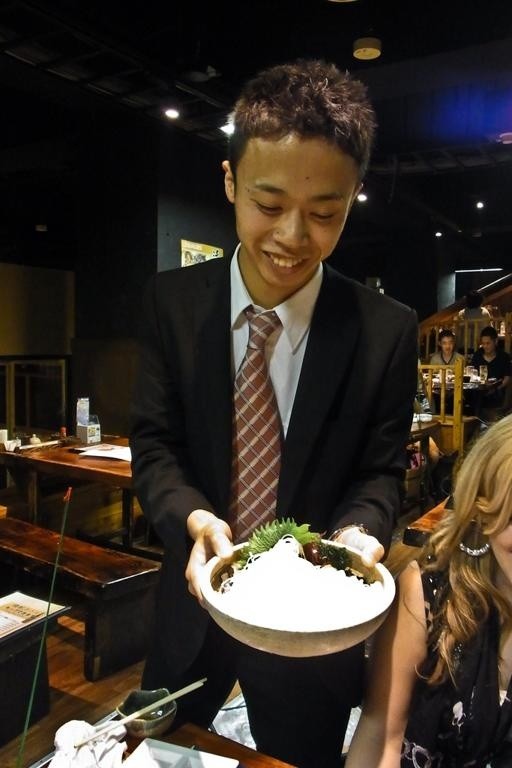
[231,307,284,550]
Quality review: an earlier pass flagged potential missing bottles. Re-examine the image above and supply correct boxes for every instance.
[60,427,66,439]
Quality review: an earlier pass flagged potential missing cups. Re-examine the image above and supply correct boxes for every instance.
[466,365,487,381]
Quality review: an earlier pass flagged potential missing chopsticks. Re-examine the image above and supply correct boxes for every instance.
[73,677,207,747]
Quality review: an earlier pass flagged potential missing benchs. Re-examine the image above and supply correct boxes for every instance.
[0,515,163,682]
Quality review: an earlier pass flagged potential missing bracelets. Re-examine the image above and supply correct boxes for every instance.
[327,522,368,541]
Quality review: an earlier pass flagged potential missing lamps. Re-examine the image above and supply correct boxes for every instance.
[353,38,381,60]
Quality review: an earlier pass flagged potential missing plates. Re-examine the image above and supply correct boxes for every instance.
[120,737,240,768]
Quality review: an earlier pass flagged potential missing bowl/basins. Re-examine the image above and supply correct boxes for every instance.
[413,413,432,422]
[116,688,178,739]
[198,534,397,658]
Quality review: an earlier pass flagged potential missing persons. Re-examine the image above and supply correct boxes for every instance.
[422,330,466,414]
[344,412,512,768]
[124,58,423,768]
[465,326,511,422]
